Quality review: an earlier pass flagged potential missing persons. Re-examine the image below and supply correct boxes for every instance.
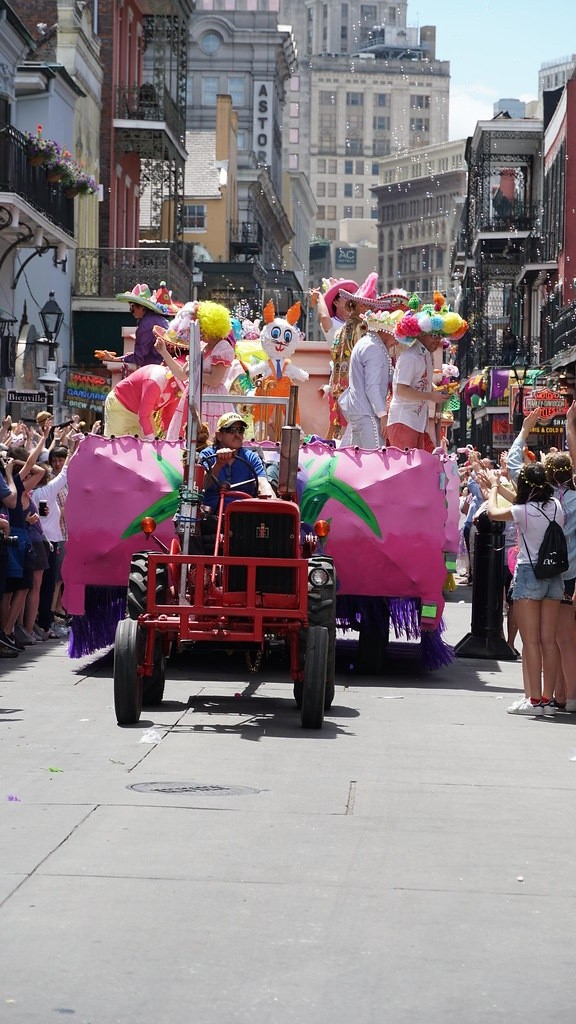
[227,319,248,389]
[99,284,174,367]
[199,413,282,556]
[386,294,467,450]
[460,401,576,715]
[309,278,359,441]
[325,272,391,442]
[154,301,235,440]
[103,356,186,440]
[338,309,405,449]
[0,412,101,658]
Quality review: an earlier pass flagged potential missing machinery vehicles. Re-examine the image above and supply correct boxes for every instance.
[112,384,340,730]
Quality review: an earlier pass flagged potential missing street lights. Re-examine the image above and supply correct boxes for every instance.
[37,290,65,449]
[511,347,529,440]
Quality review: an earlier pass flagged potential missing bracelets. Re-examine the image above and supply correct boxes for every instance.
[491,485,499,490]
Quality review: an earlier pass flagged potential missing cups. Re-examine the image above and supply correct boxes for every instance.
[39,500,47,519]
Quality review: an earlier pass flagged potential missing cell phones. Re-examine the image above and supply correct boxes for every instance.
[38,500,47,518]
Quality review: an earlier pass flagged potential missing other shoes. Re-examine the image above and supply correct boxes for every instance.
[515,649,522,658]
[457,570,472,585]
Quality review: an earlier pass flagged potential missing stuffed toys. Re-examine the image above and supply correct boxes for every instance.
[239,300,309,440]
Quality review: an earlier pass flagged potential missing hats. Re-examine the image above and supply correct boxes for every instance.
[217,412,247,432]
[116,284,262,357]
[526,451,535,461]
[316,272,469,349]
[36,411,54,426]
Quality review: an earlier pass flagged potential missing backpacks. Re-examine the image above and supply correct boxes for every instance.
[520,499,569,580]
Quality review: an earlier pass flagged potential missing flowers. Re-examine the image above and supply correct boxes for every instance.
[23,124,100,195]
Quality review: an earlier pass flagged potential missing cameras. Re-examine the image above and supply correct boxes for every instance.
[0,529,18,548]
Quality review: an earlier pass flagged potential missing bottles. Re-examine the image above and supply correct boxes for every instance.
[434,369,443,373]
[433,382,461,394]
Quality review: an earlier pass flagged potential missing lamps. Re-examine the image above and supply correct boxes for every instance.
[56,243,67,261]
[9,207,20,228]
[33,227,45,248]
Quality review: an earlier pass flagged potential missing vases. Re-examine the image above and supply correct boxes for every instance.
[63,189,79,198]
[28,154,44,167]
[47,173,62,184]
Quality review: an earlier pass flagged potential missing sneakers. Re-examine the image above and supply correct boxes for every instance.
[0,610,69,659]
[506,694,576,716]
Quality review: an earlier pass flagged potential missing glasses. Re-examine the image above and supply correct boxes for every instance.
[431,331,441,339]
[128,302,142,311]
[221,427,244,434]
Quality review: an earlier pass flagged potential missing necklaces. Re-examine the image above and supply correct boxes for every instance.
[334,315,345,324]
